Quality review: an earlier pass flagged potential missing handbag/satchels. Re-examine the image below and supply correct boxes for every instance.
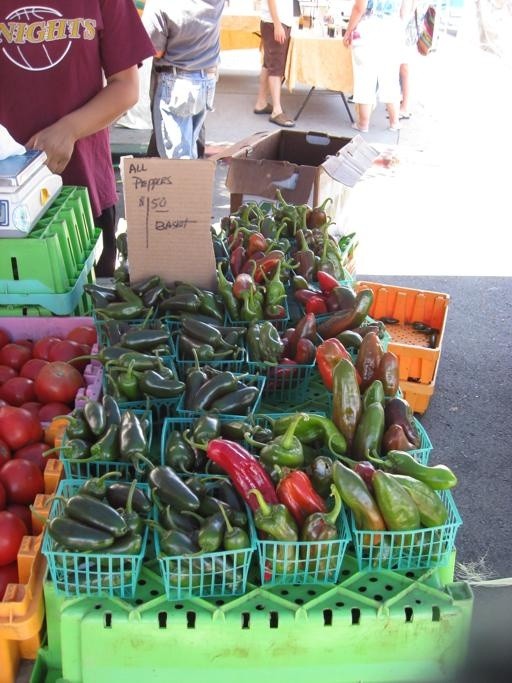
[416,6,435,57]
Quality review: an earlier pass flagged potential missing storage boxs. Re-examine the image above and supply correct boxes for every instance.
[216,123,381,224]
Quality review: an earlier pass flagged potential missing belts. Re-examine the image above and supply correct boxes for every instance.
[158,64,218,76]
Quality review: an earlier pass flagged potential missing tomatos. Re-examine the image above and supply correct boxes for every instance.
[0,325,97,604]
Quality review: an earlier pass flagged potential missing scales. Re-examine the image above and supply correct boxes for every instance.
[0,150,63,239]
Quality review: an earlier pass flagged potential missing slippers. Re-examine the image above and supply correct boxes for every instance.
[352,123,369,133]
[254,103,273,115]
[269,112,296,128]
[386,113,411,121]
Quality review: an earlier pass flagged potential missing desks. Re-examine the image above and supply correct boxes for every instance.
[284,28,358,126]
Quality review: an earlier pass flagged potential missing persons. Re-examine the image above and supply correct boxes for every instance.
[384,0,418,120]
[0,0,156,279]
[253,0,296,128]
[136,0,230,158]
[342,0,408,132]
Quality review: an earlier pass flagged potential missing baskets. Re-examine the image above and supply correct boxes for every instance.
[40,240,463,602]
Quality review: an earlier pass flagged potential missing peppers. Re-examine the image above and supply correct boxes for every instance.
[29,189,460,592]
